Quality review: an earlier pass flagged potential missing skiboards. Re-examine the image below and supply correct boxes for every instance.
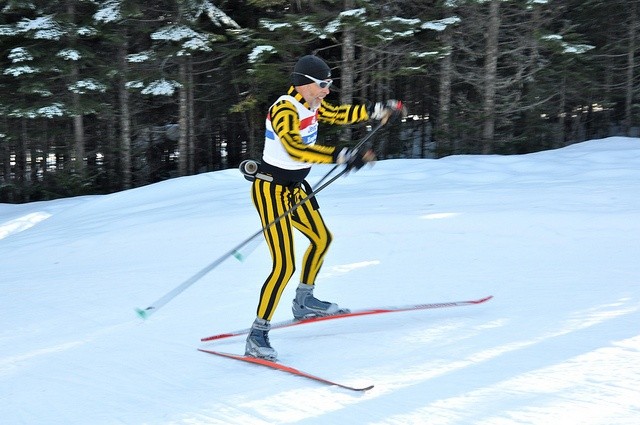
[196,295,494,391]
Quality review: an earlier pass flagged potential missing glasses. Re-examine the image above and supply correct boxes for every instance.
[289,71,333,89]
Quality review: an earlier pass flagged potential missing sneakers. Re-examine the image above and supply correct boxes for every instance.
[292,288,339,315]
[246,317,278,356]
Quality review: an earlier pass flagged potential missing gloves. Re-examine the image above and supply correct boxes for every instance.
[337,147,378,170]
[368,99,408,121]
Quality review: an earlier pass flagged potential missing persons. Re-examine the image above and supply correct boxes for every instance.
[243,53,405,361]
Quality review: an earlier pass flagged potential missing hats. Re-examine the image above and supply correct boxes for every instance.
[291,55,332,87]
